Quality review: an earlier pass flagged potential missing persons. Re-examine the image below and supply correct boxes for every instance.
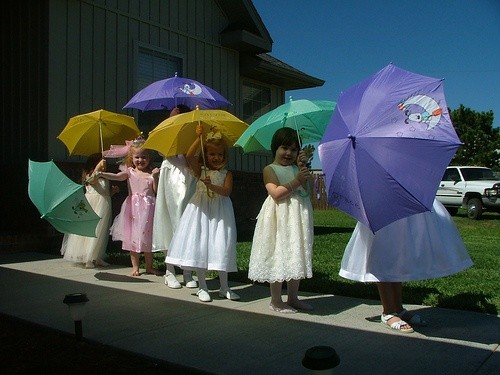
[151,104,198,288]
[338,197,474,334]
[164,132,241,302]
[60,152,120,268]
[87,138,163,277]
[248,127,315,313]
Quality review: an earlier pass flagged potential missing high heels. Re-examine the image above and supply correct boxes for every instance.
[183,272,197,287]
[164,272,181,288]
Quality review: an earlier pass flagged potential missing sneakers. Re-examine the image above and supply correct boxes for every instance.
[197,289,212,301]
[219,288,240,299]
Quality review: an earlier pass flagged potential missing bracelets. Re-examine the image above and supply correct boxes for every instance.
[288,181,295,193]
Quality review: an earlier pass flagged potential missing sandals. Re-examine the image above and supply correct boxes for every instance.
[381,312,414,333]
[399,309,426,325]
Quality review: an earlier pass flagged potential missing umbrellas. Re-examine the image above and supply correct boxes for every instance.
[318,62,465,236]
[140,104,250,199]
[121,72,233,111]
[27,158,103,238]
[55,109,145,159]
[233,95,338,198]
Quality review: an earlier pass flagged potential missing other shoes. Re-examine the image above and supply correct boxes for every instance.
[132,267,139,276]
[270,301,298,314]
[287,300,313,310]
[146,267,162,276]
[96,261,110,267]
[85,263,95,268]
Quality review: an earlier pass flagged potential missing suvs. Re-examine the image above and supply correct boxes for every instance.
[435,166,500,220]
